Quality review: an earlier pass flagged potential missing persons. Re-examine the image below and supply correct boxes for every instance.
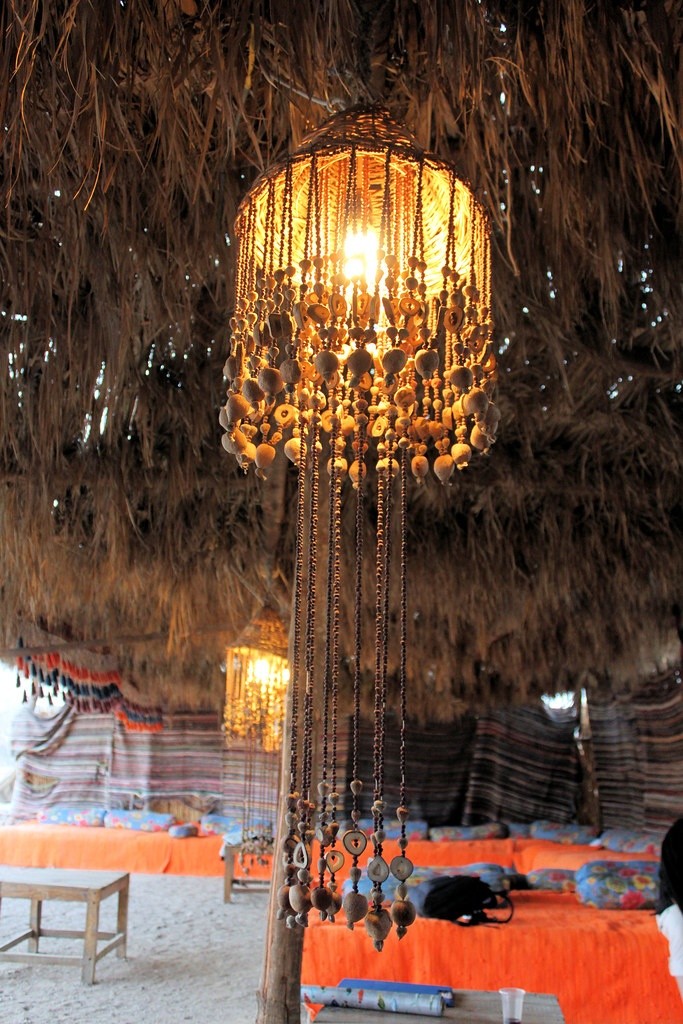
[648,819,683,1005]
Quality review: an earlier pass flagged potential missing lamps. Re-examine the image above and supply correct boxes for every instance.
[216,98,502,951]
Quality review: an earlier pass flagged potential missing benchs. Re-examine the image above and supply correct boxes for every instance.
[0,824,683,1024]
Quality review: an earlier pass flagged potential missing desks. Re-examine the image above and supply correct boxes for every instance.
[223,842,275,900]
[1,867,129,986]
[314,981,565,1024]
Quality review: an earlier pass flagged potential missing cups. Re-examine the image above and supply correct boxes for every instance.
[499,988,526,1024]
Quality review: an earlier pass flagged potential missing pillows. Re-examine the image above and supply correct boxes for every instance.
[41,804,668,914]
